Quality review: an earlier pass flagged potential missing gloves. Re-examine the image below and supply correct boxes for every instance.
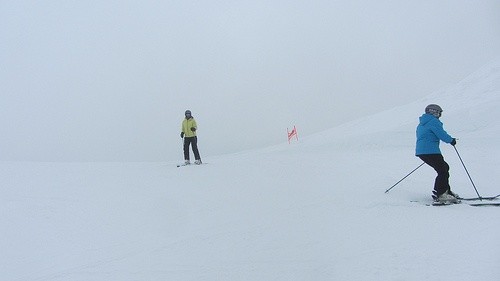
[180,132,184,138]
[451,138,456,146]
[191,127,195,131]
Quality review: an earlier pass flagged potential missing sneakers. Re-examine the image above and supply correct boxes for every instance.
[432,189,459,204]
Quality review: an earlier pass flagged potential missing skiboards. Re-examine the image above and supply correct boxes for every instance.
[410,195,500,206]
[176,161,207,168]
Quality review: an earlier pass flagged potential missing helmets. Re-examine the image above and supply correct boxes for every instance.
[425,104,443,117]
[185,110,191,116]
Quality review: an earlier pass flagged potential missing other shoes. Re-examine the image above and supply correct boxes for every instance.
[194,159,202,165]
[185,161,190,165]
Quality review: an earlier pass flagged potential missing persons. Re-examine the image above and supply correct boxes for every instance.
[180,110,202,163]
[415,104,461,202]
